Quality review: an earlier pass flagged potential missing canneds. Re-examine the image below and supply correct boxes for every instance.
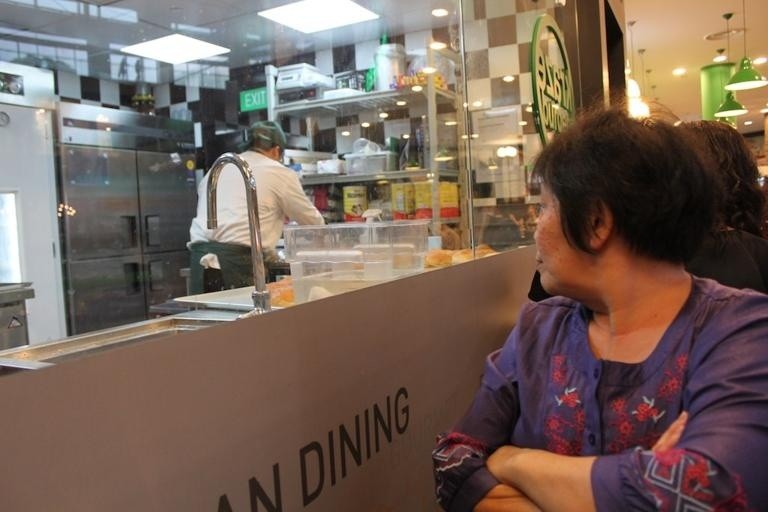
[343,186,367,223]
[391,181,460,219]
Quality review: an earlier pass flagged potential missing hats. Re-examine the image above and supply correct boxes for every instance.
[247,120,287,146]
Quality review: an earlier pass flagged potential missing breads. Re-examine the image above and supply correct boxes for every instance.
[424,244,494,267]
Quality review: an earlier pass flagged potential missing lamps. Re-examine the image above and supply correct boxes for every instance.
[725,0,767,90]
[704,14,749,119]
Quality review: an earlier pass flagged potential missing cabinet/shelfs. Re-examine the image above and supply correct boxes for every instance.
[264,36,470,251]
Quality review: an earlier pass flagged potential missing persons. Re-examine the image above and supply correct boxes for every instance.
[429,104,767,512]
[630,115,768,295]
[186,119,326,298]
[673,119,768,236]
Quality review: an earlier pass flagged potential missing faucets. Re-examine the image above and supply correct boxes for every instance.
[206,151,270,315]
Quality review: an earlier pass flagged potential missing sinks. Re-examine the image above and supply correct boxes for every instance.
[1,316,235,365]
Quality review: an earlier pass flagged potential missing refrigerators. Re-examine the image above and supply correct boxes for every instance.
[55,99,202,339]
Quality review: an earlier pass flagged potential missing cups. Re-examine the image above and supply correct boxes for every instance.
[428,235,443,252]
